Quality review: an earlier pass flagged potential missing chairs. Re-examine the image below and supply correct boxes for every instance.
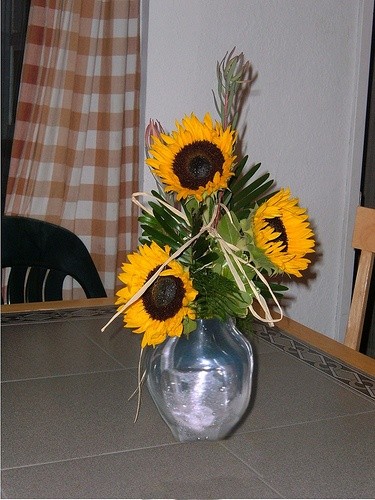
[341,204,375,359]
[2,215,105,304]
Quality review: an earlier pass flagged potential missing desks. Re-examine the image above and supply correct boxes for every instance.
[2,296,374,499]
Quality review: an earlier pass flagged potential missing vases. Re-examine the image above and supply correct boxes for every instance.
[146,313,256,442]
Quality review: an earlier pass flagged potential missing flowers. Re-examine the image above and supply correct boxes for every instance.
[112,50,317,350]
